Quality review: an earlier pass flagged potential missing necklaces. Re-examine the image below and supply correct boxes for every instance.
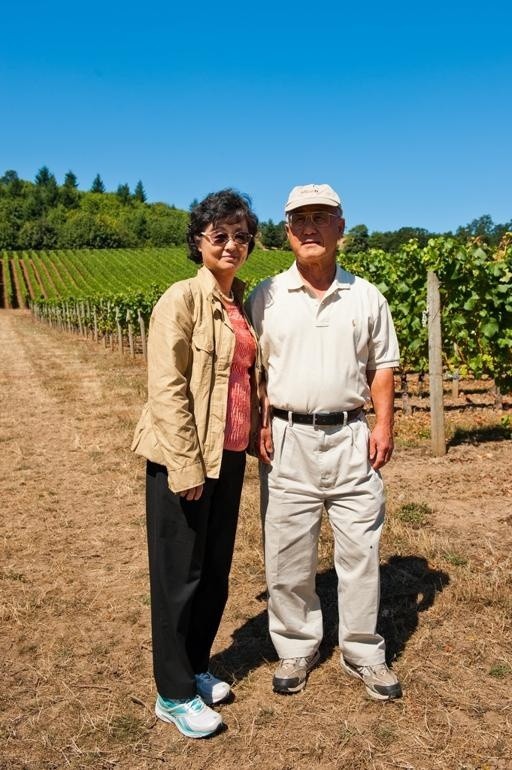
[216,287,236,302]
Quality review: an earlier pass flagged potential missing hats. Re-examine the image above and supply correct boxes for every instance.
[284,184,342,215]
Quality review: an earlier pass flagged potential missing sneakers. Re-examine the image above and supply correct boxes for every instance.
[272,650,322,695]
[339,654,402,699]
[153,691,222,740]
[196,673,231,706]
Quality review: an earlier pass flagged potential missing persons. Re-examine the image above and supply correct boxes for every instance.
[242,180,403,704]
[128,187,257,742]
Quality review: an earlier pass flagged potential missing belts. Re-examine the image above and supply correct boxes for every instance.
[268,407,365,425]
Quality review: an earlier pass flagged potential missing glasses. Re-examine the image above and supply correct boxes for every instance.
[285,212,339,228]
[197,228,254,245]
